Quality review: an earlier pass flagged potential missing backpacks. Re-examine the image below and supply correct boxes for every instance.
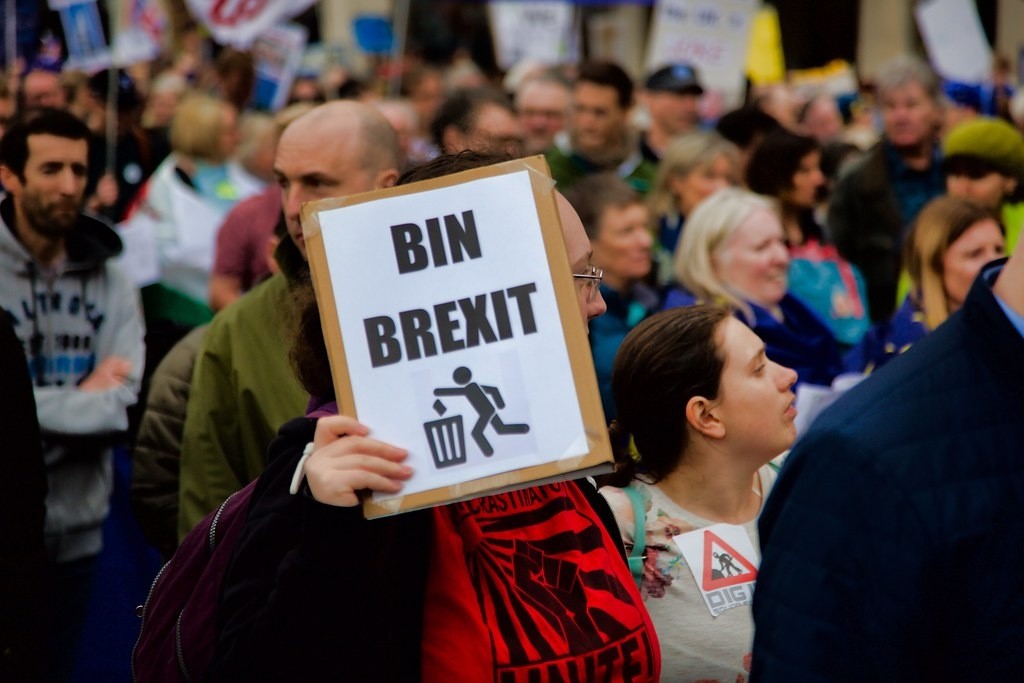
[130,398,340,683]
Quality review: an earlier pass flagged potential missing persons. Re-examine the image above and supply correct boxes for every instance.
[209,148,662,683]
[0,0,1024,683]
[590,306,797,683]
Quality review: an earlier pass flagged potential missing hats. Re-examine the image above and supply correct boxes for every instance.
[938,118,1024,176]
[645,65,703,96]
[89,69,142,115]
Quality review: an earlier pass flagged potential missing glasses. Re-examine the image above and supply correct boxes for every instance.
[573,265,603,300]
